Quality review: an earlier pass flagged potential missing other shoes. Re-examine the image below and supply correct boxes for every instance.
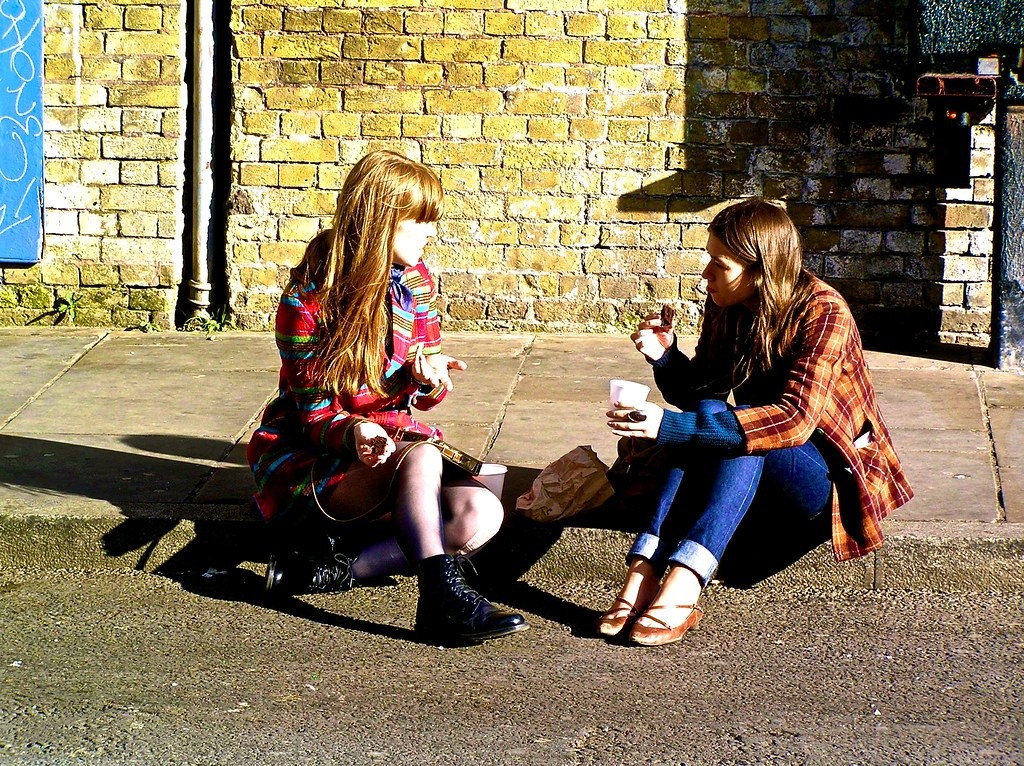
[597,602,705,648]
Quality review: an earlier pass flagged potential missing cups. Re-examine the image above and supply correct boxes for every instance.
[474,462,507,501]
[608,379,651,409]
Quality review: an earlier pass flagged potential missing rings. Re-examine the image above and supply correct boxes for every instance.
[627,409,648,423]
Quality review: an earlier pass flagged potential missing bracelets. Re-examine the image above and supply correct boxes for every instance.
[409,376,431,391]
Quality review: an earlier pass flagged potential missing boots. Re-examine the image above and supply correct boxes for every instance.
[263,548,350,601]
[415,554,529,642]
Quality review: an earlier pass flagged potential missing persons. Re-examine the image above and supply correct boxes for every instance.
[596,192,915,647]
[244,149,533,648]
